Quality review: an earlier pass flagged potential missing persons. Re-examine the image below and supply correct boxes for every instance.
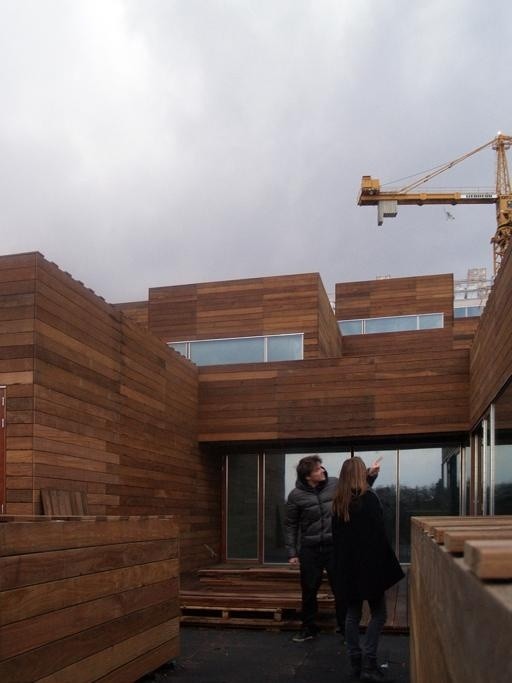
[328,457,406,683]
[285,454,383,642]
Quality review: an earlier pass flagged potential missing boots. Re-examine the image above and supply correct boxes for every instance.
[350,652,384,680]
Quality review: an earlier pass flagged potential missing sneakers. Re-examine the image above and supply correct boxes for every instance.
[292,630,313,642]
